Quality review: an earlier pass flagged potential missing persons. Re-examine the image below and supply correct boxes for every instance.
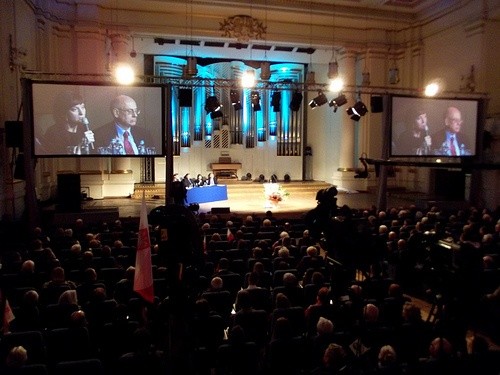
[174,172,217,188]
[0,205,500,375]
[431,106,472,155]
[90,95,155,155]
[45,92,97,155]
[398,104,432,155]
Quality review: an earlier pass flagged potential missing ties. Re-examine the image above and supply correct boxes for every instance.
[123,132,135,155]
[450,137,457,155]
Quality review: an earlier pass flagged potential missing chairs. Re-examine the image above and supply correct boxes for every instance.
[218,151,232,178]
[0,217,500,375]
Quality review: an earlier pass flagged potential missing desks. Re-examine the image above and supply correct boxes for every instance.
[209,162,243,180]
[183,184,227,205]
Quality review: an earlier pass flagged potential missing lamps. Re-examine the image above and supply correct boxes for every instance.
[303,1,316,85]
[388,0,400,84]
[360,1,371,85]
[206,85,372,122]
[327,0,339,78]
[181,0,199,79]
[218,1,267,43]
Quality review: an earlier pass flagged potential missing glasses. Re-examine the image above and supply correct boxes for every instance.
[448,118,464,124]
[117,107,141,115]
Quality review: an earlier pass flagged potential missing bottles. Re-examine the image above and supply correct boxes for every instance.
[460,144,465,155]
[138,139,145,155]
[109,139,116,155]
[443,140,449,155]
[115,139,123,155]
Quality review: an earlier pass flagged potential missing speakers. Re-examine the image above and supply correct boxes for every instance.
[57,172,83,213]
[5,121,23,147]
[429,169,465,202]
[15,155,37,180]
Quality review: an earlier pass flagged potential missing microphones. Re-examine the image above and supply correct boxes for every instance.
[424,126,430,151]
[81,117,94,150]
